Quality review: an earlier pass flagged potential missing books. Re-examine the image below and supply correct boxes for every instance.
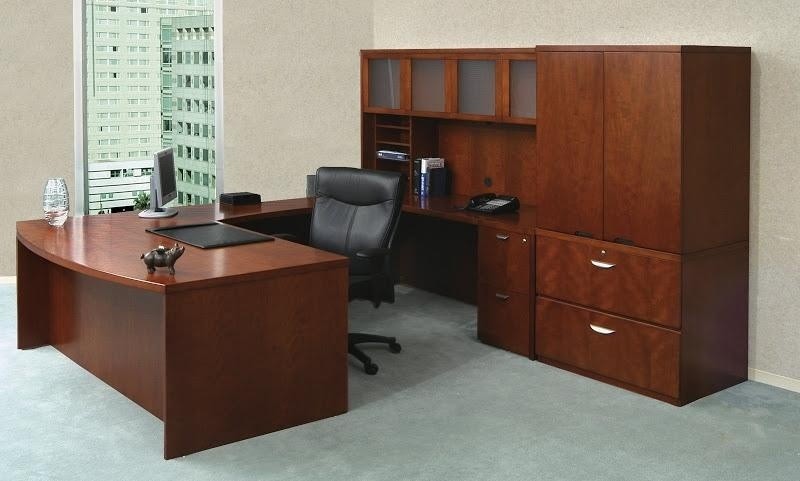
[413,157,447,194]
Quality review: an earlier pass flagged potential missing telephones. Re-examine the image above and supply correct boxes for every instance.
[452,192,519,215]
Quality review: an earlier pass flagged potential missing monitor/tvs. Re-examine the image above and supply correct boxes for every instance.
[137,146,178,219]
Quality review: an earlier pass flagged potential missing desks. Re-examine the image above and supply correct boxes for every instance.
[15,196,348,460]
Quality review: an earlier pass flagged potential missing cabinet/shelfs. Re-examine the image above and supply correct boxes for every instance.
[534,46,751,255]
[535,228,748,407]
[360,45,535,362]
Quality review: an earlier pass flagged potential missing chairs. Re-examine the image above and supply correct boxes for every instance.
[273,166,409,375]
